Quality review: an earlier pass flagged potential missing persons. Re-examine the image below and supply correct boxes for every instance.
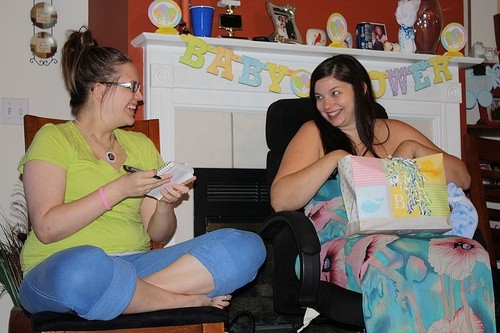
[276,15,295,39]
[17,26,267,321]
[270,54,497,333]
[371,25,386,51]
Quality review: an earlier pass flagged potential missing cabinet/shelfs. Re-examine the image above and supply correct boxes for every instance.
[465,122,500,268]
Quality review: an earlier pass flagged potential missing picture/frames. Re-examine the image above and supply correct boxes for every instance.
[265,0,302,46]
[371,22,388,43]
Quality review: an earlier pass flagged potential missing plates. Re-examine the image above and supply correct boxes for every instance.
[326,12,348,41]
[147,0,182,28]
[440,22,468,51]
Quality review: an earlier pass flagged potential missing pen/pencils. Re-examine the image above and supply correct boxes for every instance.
[122,164,162,180]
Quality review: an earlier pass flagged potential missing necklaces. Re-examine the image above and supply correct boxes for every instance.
[74,119,116,162]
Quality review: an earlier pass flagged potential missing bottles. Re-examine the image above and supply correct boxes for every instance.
[413,0,443,54]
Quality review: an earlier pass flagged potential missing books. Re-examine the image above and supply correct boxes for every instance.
[146,161,194,201]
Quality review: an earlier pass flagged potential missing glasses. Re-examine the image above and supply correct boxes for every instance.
[91,80,141,92]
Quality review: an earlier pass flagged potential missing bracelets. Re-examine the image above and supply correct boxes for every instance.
[98,187,112,211]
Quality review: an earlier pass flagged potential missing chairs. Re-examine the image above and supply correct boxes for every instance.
[257,97,487,333]
[8,115,223,333]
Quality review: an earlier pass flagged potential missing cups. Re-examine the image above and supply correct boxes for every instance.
[189,5,218,37]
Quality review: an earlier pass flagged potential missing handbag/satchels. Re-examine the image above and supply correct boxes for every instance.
[338,152,453,234]
